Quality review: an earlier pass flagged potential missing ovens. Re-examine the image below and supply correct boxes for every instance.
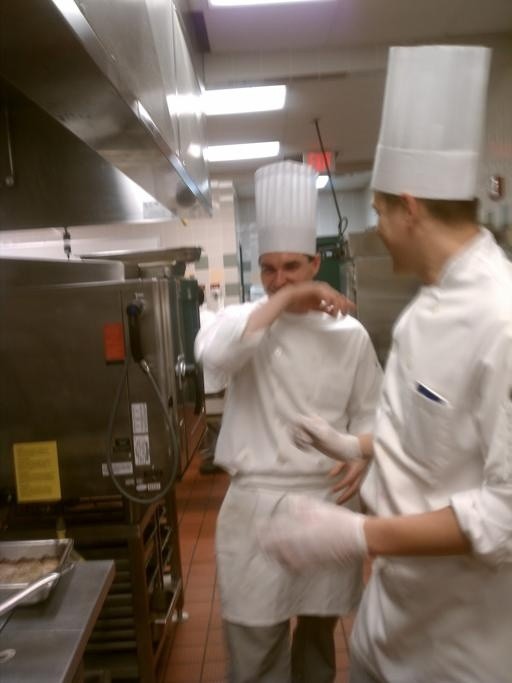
[1,273,209,683]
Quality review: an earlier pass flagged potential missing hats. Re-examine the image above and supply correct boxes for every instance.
[252,161,318,258]
[371,44,492,200]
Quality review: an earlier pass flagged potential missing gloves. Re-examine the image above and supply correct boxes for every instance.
[293,416,362,462]
[276,497,366,570]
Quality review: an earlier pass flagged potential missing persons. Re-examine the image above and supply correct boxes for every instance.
[253,187,510,682]
[192,250,381,682]
